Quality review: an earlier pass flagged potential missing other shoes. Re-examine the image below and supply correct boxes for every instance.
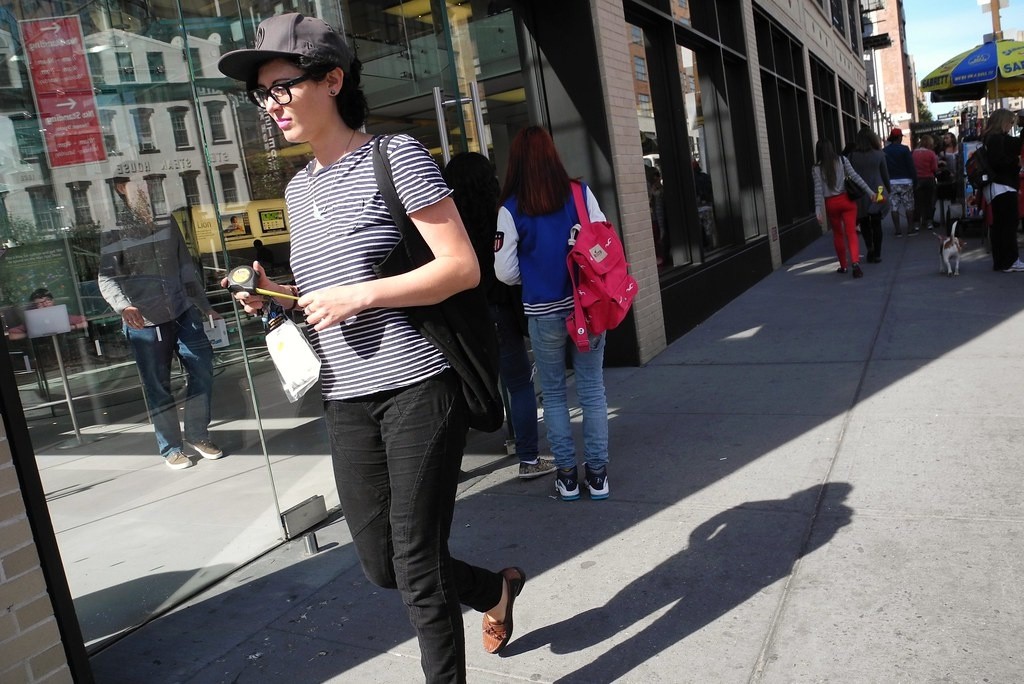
[837,267,848,274]
[913,226,919,231]
[866,254,881,263]
[850,262,864,278]
[907,230,918,237]
[894,232,903,238]
[926,222,933,230]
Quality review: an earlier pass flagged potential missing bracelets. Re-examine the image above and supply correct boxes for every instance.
[284,285,298,310]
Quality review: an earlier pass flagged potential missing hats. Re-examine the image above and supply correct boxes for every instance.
[691,159,701,172]
[886,128,905,142]
[218,12,352,76]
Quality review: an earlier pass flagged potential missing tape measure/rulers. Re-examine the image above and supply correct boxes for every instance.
[226,265,300,300]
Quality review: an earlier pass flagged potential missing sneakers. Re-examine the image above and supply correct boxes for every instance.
[482,566,526,654]
[519,458,557,479]
[583,462,609,501]
[168,448,193,469]
[185,436,223,458]
[1002,257,1024,273]
[554,465,581,501]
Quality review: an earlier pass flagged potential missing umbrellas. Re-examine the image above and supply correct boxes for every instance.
[921,31,1024,119]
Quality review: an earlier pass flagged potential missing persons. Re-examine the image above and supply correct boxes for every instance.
[224,216,244,233]
[844,128,889,263]
[492,127,613,501]
[883,128,920,238]
[7,289,87,340]
[976,109,1024,273]
[434,152,559,479]
[910,133,959,231]
[693,160,712,205]
[812,139,877,277]
[222,12,525,684]
[643,159,668,269]
[97,161,226,469]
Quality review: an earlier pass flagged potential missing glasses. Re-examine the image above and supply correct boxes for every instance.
[245,68,322,110]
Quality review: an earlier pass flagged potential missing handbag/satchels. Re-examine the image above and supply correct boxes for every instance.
[841,154,865,202]
[370,131,523,431]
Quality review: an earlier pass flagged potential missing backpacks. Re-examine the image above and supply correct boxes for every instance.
[564,178,638,354]
[965,146,993,191]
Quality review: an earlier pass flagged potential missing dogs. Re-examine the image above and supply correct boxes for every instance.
[942,221,967,276]
